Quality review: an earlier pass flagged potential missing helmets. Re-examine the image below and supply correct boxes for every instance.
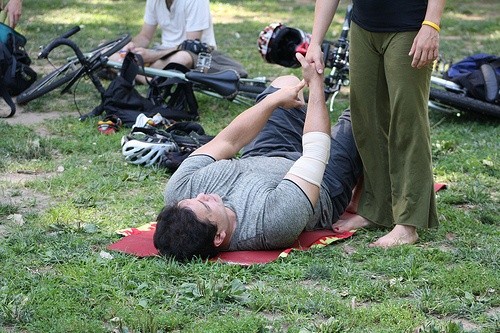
[122,132,180,168]
[147,62,192,111]
[257,22,310,69]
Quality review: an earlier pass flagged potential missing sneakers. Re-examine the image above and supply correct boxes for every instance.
[98,114,121,134]
[133,113,175,135]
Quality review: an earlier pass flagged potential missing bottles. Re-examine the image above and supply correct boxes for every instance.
[146,121,155,129]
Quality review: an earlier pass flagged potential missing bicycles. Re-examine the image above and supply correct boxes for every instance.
[14,24,276,124]
[259,1,500,123]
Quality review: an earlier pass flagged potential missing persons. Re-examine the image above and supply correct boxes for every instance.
[306,0,446,247]
[0,0,22,30]
[154,53,365,262]
[98,0,217,87]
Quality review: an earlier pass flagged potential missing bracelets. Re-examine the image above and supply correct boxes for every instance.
[422,20,440,33]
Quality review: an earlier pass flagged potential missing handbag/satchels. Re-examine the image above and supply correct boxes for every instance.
[0,21,38,95]
[103,74,159,126]
[442,52,500,101]
